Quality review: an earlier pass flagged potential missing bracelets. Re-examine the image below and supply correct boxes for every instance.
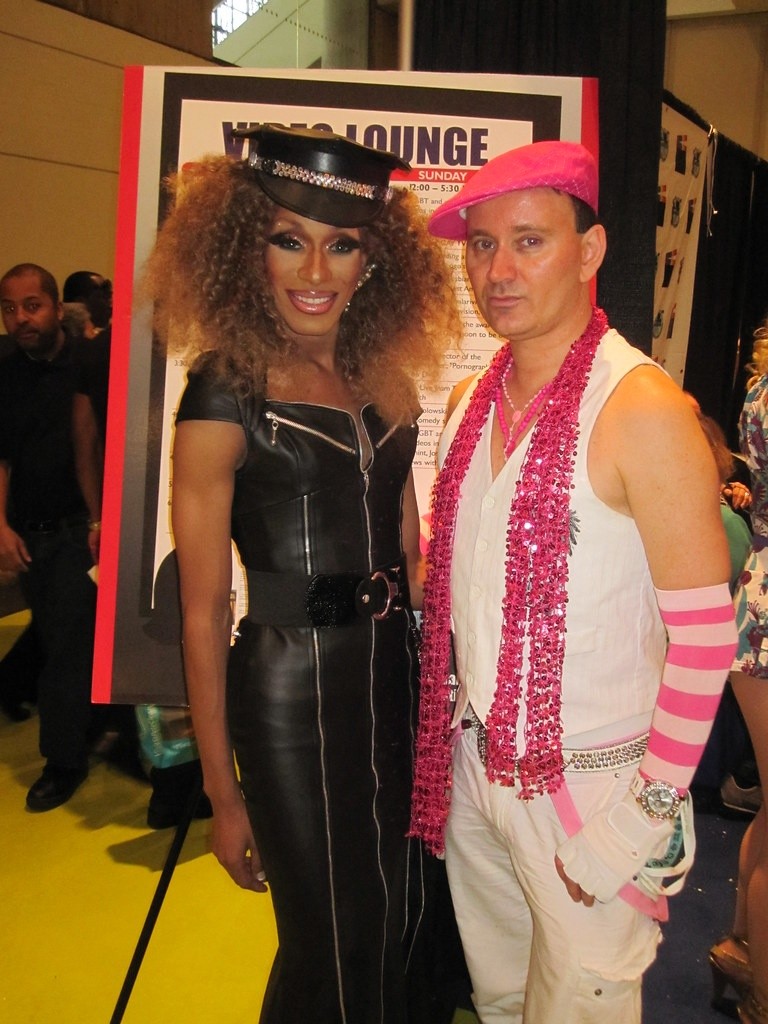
[88,521,101,531]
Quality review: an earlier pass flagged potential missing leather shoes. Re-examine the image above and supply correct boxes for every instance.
[27,755,87,810]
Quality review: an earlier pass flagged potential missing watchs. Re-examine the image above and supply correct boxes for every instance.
[630,769,684,821]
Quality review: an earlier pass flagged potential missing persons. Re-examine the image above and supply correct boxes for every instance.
[138,123,462,1024]
[0,263,136,813]
[645,336,768,1024]
[423,142,738,1024]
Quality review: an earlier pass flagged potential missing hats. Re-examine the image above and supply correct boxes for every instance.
[428,141,597,242]
[230,126,410,226]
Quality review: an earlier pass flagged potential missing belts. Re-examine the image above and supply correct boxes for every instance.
[16,515,90,536]
[469,714,648,773]
[246,553,407,630]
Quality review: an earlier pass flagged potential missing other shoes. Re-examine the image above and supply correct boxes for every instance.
[0,664,31,722]
[712,774,763,818]
[144,759,212,830]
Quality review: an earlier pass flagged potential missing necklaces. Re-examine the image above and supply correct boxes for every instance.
[495,356,553,461]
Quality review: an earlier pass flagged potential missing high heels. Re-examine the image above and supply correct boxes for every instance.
[709,930,768,1023]
